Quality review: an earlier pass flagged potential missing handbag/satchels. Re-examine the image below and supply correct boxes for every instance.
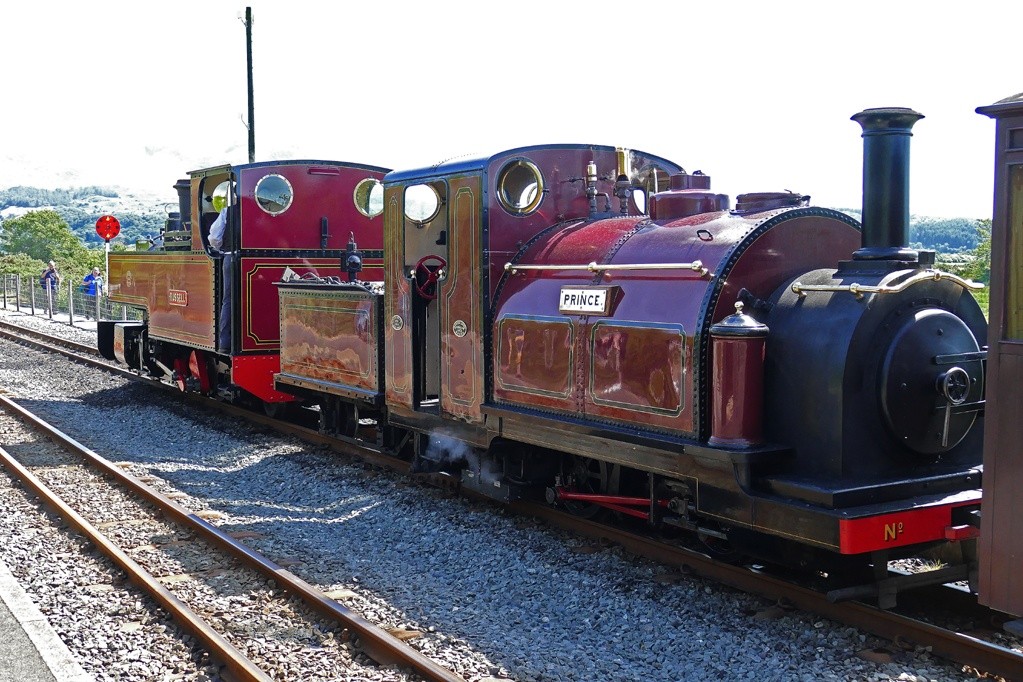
[40,270,46,287]
[79,275,91,294]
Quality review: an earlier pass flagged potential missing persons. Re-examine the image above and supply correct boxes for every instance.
[41,261,59,315]
[81,267,103,321]
[208,185,239,353]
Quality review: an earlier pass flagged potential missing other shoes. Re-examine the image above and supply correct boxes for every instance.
[53,312,57,315]
[44,311,47,314]
[94,318,97,321]
[218,348,231,354]
[86,315,89,320]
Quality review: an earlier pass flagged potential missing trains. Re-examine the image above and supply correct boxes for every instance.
[98,106,988,610]
